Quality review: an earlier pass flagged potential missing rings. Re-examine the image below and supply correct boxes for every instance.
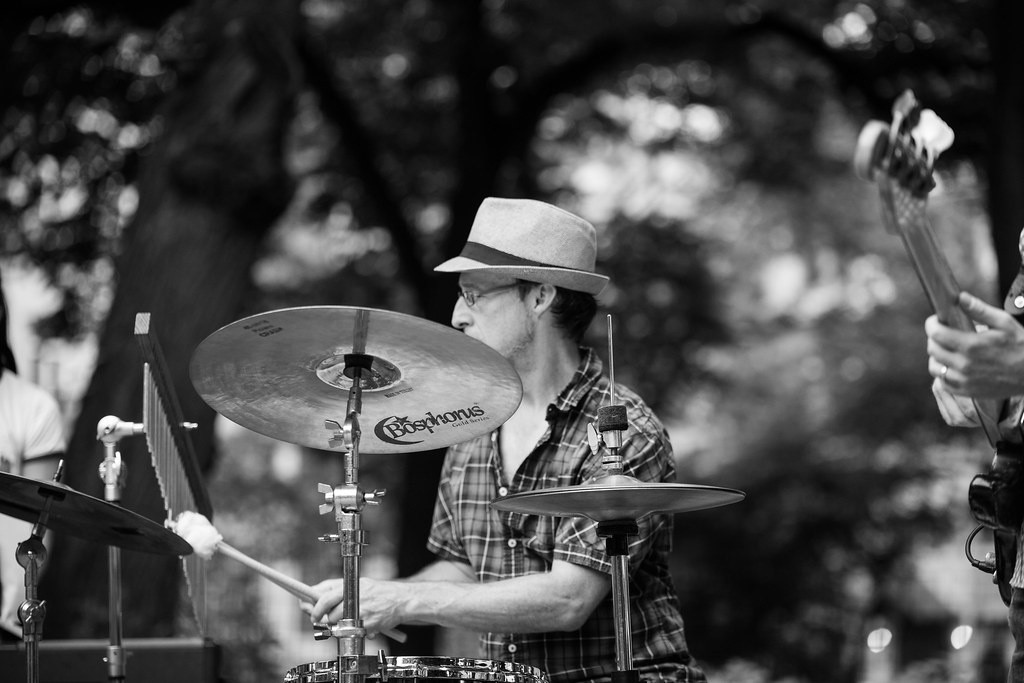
[941,366,948,382]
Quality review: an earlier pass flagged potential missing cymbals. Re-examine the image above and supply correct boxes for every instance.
[184,304,525,456]
[489,454,748,524]
[1,456,196,562]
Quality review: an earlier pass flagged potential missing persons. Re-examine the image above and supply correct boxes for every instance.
[301,196,706,683]
[0,286,70,637]
[927,267,1024,683]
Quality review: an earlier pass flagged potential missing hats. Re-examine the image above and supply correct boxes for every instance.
[433,197,610,295]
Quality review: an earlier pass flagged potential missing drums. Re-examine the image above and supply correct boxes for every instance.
[283,654,550,683]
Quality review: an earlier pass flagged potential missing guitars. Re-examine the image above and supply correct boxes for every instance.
[853,80,1024,613]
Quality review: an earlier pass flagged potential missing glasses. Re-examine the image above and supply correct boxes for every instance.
[457,282,521,306]
